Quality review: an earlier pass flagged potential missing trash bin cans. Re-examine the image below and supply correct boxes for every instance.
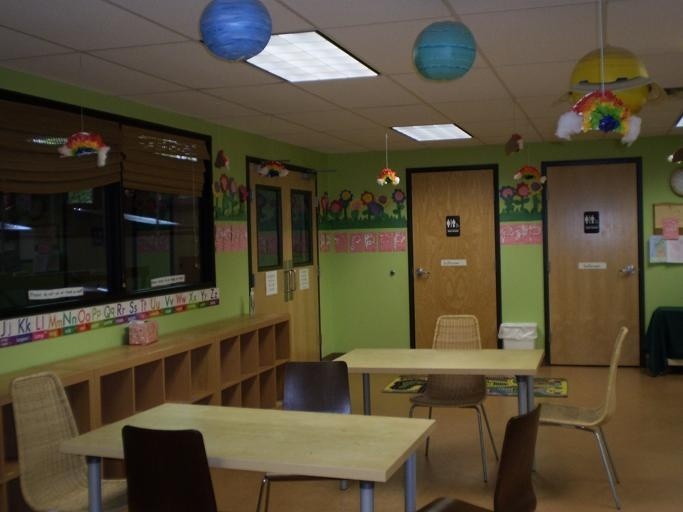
[498,323,538,349]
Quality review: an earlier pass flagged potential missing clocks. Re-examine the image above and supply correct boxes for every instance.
[669,167,683,197]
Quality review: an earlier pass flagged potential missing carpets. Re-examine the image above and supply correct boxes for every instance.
[381,376,568,397]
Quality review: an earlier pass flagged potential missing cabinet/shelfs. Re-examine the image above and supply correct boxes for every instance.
[0,314,291,512]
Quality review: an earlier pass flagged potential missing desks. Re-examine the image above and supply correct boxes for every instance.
[332,348,546,490]
[60,403,437,512]
[647,307,683,377]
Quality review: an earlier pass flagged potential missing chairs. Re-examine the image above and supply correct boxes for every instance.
[256,361,352,512]
[538,326,630,510]
[412,403,544,512]
[11,370,128,512]
[409,315,499,482]
[122,424,220,512]
